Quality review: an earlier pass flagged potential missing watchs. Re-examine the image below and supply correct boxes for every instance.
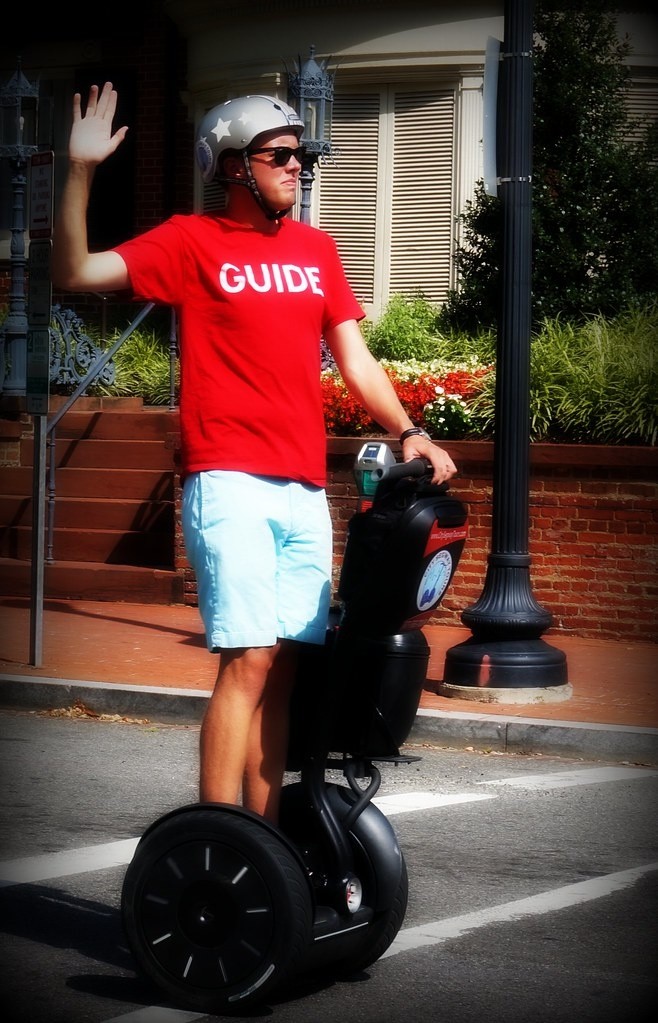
[399,427,432,446]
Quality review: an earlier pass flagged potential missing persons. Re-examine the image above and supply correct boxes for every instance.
[54,82,457,823]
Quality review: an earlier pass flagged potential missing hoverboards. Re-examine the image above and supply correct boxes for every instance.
[115,456,470,1018]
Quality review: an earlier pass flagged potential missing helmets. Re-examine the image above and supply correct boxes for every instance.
[196,94,305,182]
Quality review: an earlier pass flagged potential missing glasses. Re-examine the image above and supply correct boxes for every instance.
[245,146,305,167]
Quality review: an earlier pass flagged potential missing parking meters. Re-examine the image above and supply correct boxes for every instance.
[352,442,397,514]
